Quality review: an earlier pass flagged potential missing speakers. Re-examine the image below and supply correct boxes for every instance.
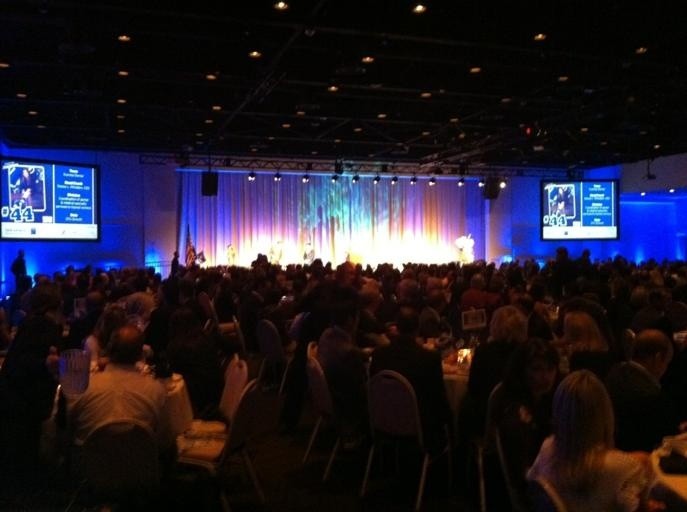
[482,176,501,199]
[202,173,218,196]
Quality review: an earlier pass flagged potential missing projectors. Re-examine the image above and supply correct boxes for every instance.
[641,173,656,181]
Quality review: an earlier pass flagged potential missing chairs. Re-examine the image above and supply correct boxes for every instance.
[232,315,248,355]
[358,370,431,510]
[182,358,249,438]
[534,474,570,512]
[258,318,295,393]
[301,341,365,482]
[42,383,62,434]
[474,382,518,512]
[176,378,285,510]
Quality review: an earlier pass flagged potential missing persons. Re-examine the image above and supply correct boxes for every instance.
[0,240,687,511]
[550,187,572,227]
[10,168,40,205]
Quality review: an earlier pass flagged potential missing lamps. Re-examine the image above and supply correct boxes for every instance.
[247,171,507,190]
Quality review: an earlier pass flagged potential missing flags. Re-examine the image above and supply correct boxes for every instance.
[185,223,198,265]
